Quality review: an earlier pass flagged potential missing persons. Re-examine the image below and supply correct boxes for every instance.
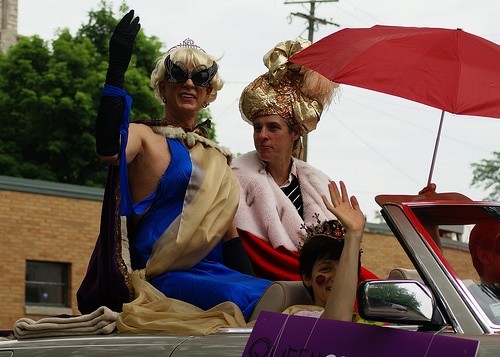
[229,84,384,315]
[95,8,273,326]
[416,184,500,301]
[281,181,366,323]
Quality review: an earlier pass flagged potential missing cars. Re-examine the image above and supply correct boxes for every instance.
[0,195,500,357]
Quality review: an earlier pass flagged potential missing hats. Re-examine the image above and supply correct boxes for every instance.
[239,36,337,137]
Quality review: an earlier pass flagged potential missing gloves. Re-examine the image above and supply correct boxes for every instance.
[96,9,141,157]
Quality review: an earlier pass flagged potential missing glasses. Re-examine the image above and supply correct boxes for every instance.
[164,54,219,88]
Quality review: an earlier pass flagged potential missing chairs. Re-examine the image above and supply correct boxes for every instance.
[249,280,316,330]
[389,265,430,285]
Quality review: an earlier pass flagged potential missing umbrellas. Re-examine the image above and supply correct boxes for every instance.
[286,24,500,188]
